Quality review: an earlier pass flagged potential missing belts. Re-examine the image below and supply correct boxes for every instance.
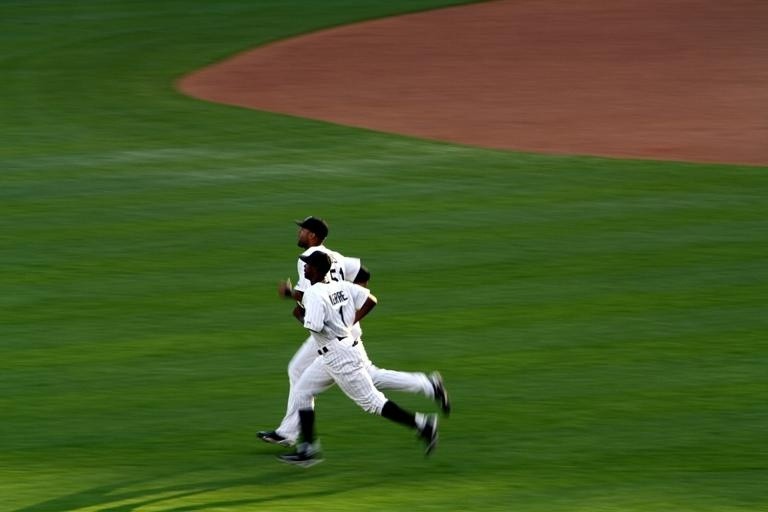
[317,337,346,355]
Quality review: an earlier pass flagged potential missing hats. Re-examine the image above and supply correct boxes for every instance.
[294,215,328,237]
[299,252,331,273]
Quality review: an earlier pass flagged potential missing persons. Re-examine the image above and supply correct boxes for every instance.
[276,250,438,465]
[255,216,450,447]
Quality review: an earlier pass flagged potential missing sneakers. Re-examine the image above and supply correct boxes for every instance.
[427,370,450,412]
[418,413,439,455]
[256,430,292,448]
